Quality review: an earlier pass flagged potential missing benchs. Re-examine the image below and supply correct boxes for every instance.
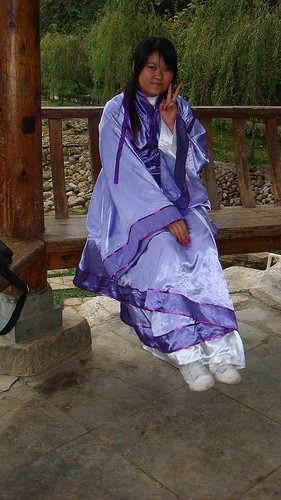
[41,106,281,270]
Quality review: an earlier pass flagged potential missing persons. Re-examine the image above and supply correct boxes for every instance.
[72,35,246,391]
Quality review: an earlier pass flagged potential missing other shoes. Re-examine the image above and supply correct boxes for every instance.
[208,362,241,384]
[177,359,217,391]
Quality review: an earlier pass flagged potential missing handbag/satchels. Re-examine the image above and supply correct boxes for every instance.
[0,240,15,269]
